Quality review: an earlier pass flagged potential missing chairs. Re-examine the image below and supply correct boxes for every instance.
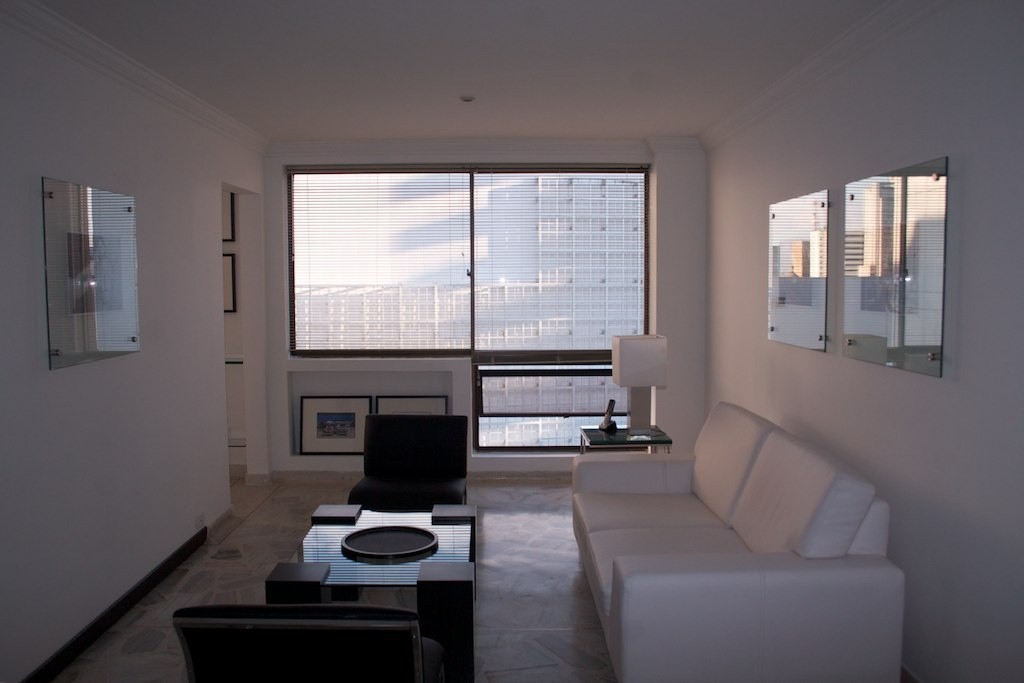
[172,601,448,683]
[347,416,468,513]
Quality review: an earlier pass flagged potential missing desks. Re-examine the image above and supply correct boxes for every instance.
[580,428,672,459]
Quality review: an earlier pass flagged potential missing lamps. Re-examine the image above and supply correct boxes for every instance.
[611,334,668,435]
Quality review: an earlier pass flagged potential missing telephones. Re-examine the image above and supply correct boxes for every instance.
[599,399,616,432]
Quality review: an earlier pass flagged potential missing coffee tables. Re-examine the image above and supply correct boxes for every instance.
[265,504,476,682]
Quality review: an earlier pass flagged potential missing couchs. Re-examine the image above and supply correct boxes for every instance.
[569,402,905,683]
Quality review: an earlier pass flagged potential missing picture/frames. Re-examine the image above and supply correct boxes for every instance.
[223,253,237,313]
[222,193,236,242]
[300,395,373,455]
[376,395,448,416]
